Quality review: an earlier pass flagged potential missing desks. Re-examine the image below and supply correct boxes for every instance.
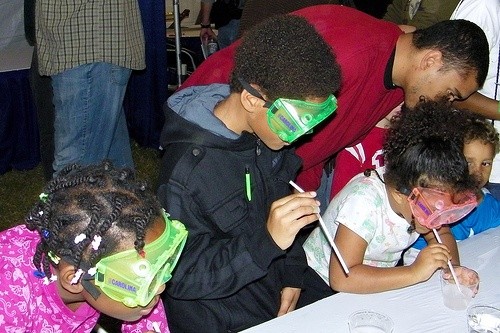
[239,224,500,333]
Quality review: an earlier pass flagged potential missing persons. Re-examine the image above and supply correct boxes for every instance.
[0,0,40,174]
[177,4,489,204]
[0,158,188,333]
[126,0,169,149]
[384,0,461,35]
[35,0,145,176]
[450,0,500,196]
[402,113,500,267]
[166,0,217,42]
[158,15,341,333]
[303,97,482,295]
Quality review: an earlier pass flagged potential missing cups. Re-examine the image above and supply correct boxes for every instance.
[347,309,395,333]
[439,265,480,311]
[465,303,500,333]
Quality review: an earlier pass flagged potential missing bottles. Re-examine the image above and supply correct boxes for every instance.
[207,24,218,56]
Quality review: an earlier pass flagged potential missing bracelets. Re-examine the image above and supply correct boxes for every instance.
[201,23,210,28]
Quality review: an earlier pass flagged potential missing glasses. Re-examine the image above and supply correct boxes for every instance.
[235,73,338,143]
[399,183,478,230]
[65,209,188,307]
